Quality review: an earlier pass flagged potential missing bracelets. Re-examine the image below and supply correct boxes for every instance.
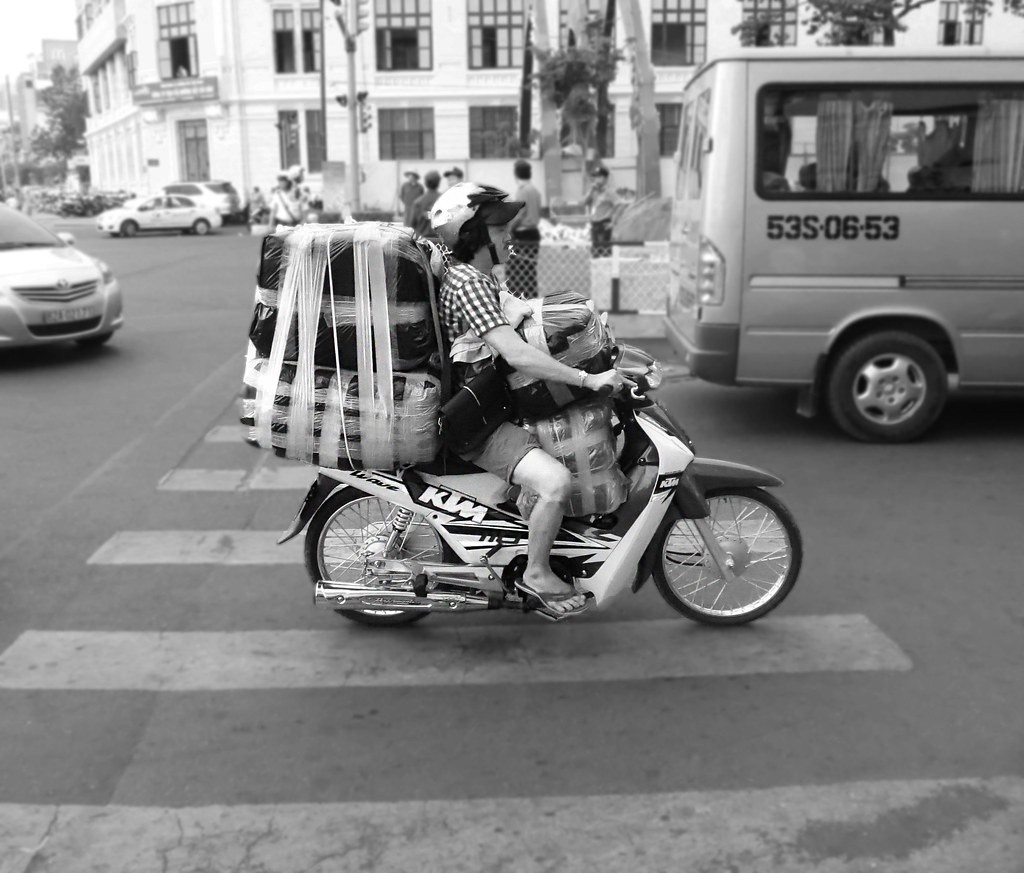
[579,368,587,389]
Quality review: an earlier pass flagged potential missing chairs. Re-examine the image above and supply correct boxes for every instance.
[759,147,971,196]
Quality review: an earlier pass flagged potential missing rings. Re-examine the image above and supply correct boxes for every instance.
[618,383,623,386]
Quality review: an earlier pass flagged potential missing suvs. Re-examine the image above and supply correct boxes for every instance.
[146,181,242,223]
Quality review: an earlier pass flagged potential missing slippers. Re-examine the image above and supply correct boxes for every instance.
[515,578,589,616]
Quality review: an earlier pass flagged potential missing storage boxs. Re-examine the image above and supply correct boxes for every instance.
[238,221,632,518]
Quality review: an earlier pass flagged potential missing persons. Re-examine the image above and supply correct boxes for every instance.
[585,167,626,257]
[401,165,466,235]
[251,166,314,233]
[430,181,638,616]
[507,160,541,298]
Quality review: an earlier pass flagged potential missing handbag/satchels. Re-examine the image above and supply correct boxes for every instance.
[436,365,517,456]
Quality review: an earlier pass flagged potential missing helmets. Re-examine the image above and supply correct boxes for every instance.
[430,182,510,253]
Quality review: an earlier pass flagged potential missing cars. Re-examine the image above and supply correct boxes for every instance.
[0,202,124,356]
[96,194,222,238]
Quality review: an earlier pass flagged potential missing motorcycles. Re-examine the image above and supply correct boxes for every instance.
[274,311,804,627]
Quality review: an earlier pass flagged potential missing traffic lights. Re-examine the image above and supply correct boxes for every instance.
[357,0,368,32]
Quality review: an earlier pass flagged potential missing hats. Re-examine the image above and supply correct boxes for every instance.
[462,198,525,232]
[589,167,609,178]
[404,170,419,180]
[443,167,463,178]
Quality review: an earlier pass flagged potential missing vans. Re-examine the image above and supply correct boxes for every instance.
[663,47,1024,443]
